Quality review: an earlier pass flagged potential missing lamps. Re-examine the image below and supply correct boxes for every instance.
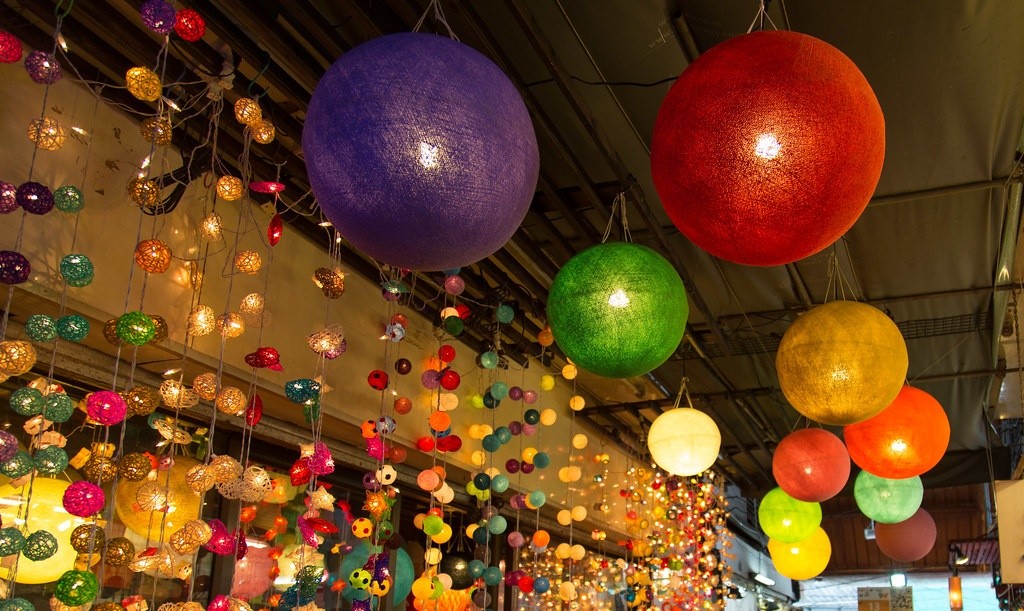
[544,185,688,382]
[645,340,723,478]
[649,1,890,274]
[300,0,542,280]
[756,243,952,583]
